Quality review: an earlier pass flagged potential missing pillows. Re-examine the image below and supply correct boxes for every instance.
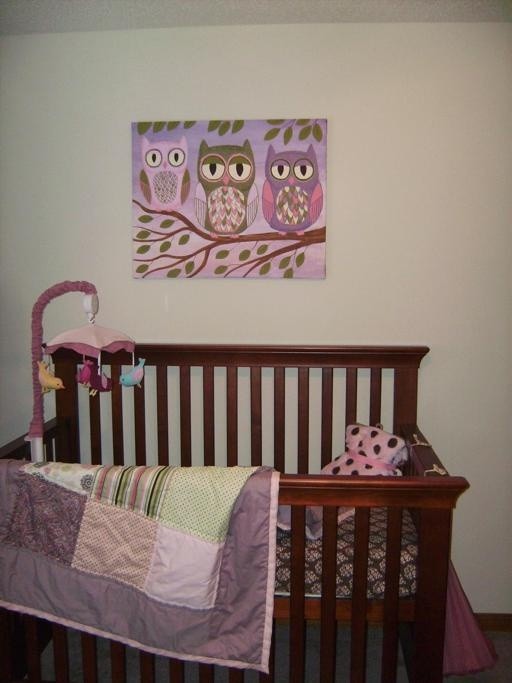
[306,423,408,523]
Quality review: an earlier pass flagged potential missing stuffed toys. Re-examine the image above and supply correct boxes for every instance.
[24,278,148,464]
[275,419,410,543]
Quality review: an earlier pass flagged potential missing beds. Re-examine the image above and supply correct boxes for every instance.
[0,344,471,683]
